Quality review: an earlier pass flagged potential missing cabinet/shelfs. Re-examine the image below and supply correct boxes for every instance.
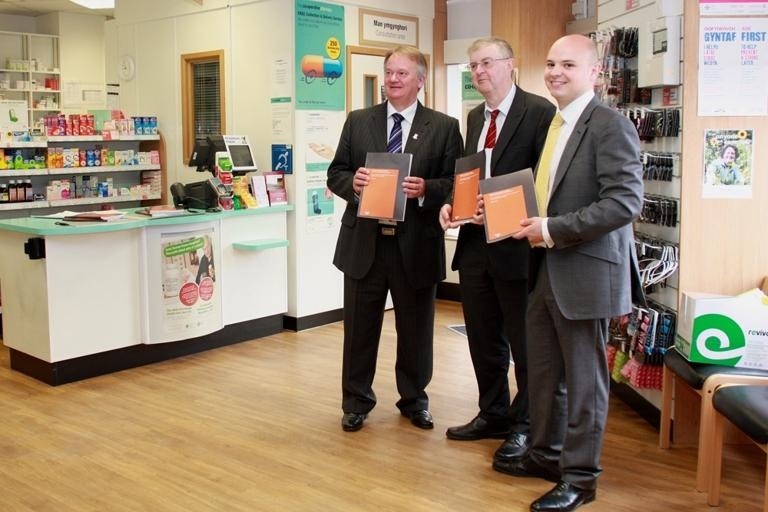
[1,30,62,134]
[1,131,167,211]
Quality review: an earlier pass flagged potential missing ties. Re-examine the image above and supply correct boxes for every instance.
[385,113,405,154]
[483,108,499,148]
[534,112,565,218]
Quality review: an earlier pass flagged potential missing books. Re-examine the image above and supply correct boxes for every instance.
[357,152,413,221]
[448,149,492,228]
[478,167,541,244]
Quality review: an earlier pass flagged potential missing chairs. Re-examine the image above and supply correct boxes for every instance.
[657,275,767,492]
[706,380,767,510]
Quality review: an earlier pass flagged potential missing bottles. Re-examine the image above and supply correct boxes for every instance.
[1,179,33,202]
[312,191,321,215]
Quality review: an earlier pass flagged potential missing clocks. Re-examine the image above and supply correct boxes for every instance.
[117,55,135,80]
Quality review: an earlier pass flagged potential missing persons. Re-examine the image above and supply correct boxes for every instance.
[707,145,745,186]
[196,235,216,286]
[493,35,644,512]
[439,37,556,464]
[326,44,465,432]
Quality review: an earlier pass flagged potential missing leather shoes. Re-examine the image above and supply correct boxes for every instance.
[493,427,570,483]
[340,400,368,432]
[400,400,434,431]
[446,414,512,441]
[529,479,598,510]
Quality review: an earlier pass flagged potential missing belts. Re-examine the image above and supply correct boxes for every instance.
[377,224,405,238]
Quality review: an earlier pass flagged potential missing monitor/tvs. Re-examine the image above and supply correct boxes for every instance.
[189,136,226,174]
[226,143,258,172]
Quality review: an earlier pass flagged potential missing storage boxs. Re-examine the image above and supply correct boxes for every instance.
[674,288,767,373]
